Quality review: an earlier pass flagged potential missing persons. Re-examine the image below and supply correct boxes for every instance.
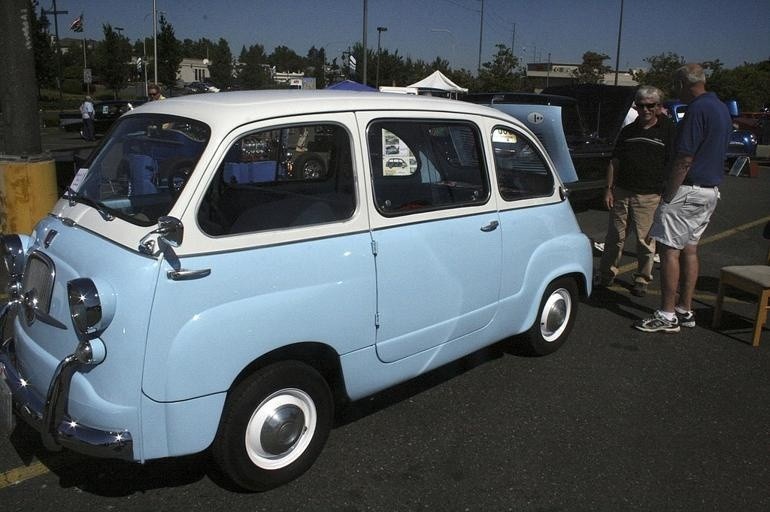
[80,94,97,143]
[632,61,733,332]
[593,87,672,265]
[592,85,674,295]
[147,85,173,130]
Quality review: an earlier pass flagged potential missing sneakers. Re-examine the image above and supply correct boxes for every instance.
[674,306,697,328]
[633,309,680,333]
[593,271,614,288]
[631,280,648,298]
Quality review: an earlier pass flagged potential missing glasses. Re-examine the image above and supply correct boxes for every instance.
[634,103,658,109]
[149,92,159,96]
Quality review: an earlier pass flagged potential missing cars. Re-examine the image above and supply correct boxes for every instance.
[663,102,758,160]
[0,89,593,493]
[462,91,615,213]
[56,99,147,132]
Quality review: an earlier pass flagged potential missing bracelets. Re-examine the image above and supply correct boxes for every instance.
[605,184,614,190]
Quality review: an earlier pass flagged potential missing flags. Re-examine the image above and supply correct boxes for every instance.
[67,15,85,34]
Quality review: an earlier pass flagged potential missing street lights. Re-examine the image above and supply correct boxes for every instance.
[143,9,168,96]
[68,14,90,96]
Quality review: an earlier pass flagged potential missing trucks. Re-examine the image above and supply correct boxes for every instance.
[288,76,317,89]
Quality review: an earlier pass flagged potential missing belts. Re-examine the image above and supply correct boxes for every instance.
[681,182,719,189]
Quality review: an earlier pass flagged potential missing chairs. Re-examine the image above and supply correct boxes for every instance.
[710,219,770,347]
